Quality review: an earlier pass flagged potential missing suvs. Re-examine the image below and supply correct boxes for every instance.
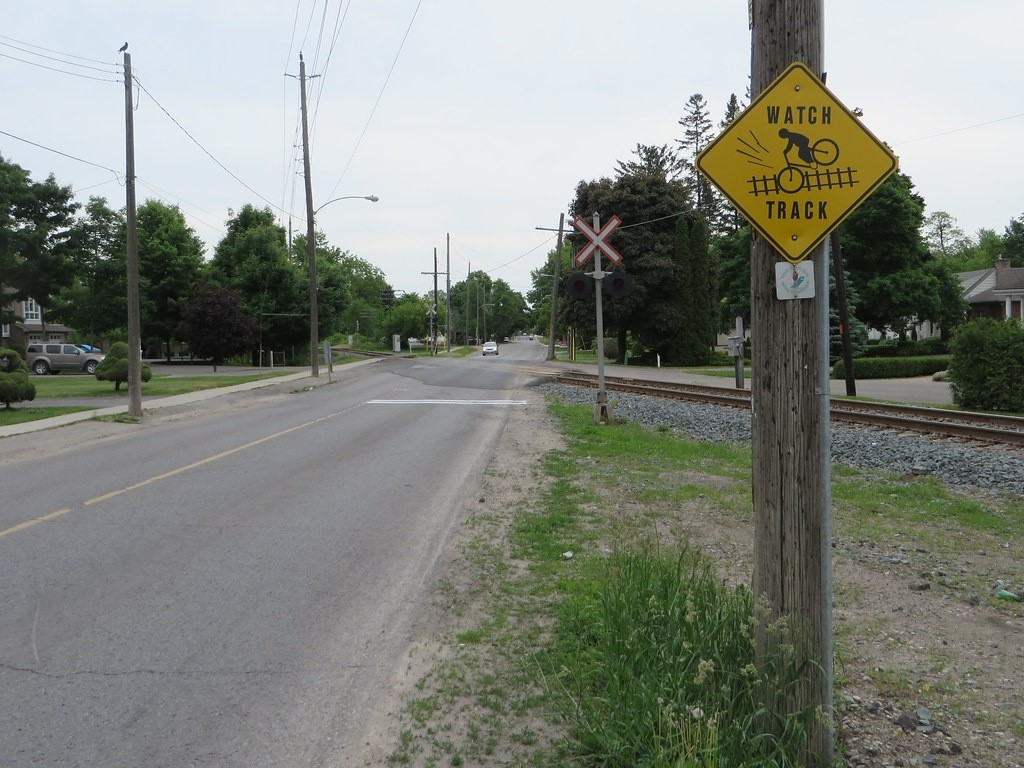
[26,344,106,374]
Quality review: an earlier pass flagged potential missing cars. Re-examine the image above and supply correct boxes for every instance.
[523,333,527,336]
[529,334,533,340]
[504,337,510,342]
[467,336,480,345]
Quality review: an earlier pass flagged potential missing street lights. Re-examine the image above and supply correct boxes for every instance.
[476,302,503,346]
[540,273,560,360]
[482,342,499,356]
[307,195,379,377]
[466,275,477,345]
